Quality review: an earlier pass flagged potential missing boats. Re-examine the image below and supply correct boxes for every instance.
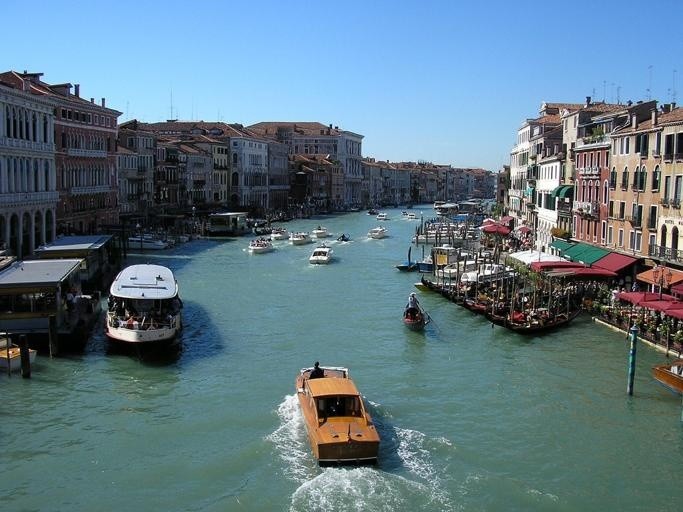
[295,366,380,469]
[129,229,191,251]
[365,208,391,221]
[400,196,588,336]
[247,238,276,255]
[311,224,334,239]
[0,332,37,372]
[286,232,315,246]
[651,358,683,394]
[104,261,191,352]
[309,243,337,265]
[366,225,390,242]
[271,228,290,242]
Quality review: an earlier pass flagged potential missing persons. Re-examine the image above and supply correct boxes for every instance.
[313,253,317,256]
[318,226,321,229]
[456,235,679,330]
[321,253,326,255]
[404,292,419,320]
[66,290,75,318]
[107,293,183,331]
[309,361,324,379]
[251,201,309,246]
[341,234,345,238]
